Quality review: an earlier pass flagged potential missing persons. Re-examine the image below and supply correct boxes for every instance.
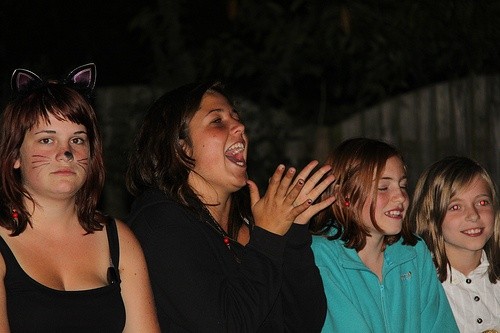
[124,78,337,332]
[2,79,162,333]
[309,136,461,332]
[408,149,500,333]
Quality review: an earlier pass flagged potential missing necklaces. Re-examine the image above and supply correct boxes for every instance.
[200,202,234,254]
